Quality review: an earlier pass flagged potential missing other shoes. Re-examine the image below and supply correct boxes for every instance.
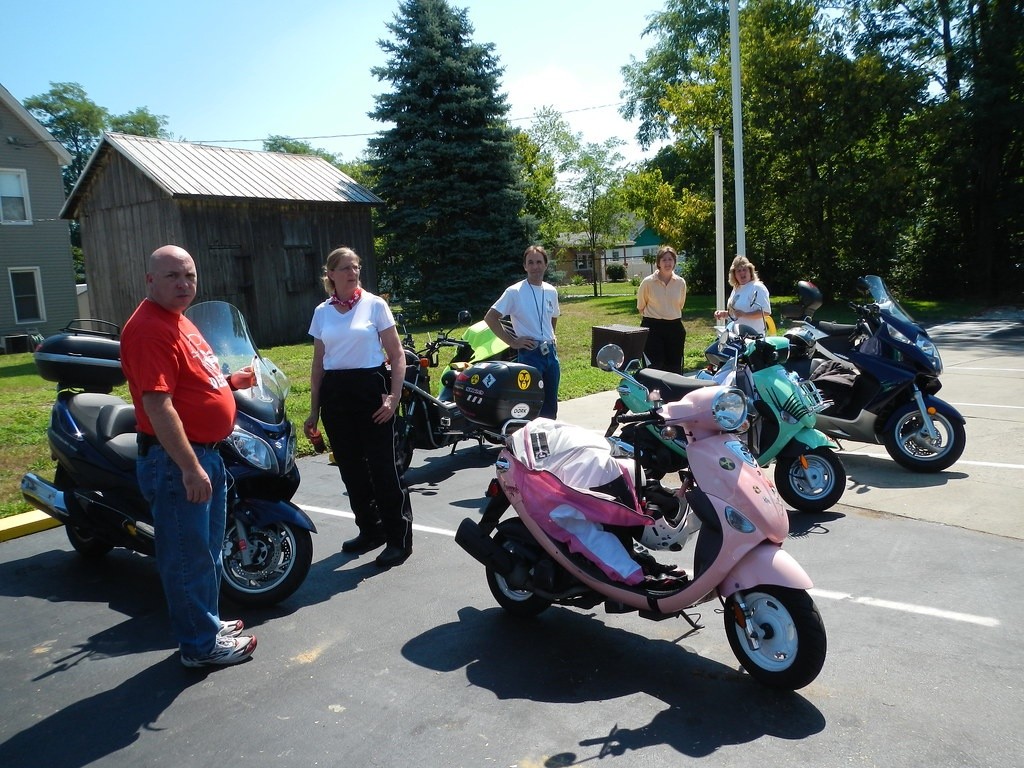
[376,545,412,566]
[343,530,386,552]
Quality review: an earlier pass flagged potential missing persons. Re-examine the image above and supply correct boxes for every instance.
[304,246,412,563]
[713,254,772,338]
[636,246,687,375]
[485,245,561,420]
[120,245,256,666]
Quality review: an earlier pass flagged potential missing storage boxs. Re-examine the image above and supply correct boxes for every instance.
[590,324,649,371]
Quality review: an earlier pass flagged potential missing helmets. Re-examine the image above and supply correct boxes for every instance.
[782,327,816,361]
[635,484,700,552]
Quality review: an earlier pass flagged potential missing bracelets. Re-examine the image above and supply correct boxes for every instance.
[226,375,238,391]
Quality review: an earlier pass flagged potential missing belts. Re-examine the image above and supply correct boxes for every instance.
[137,433,222,451]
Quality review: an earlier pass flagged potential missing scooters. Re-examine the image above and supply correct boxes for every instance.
[455,325,828,691]
[590,291,846,512]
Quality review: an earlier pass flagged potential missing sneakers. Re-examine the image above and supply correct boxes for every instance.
[216,619,245,639]
[181,633,257,667]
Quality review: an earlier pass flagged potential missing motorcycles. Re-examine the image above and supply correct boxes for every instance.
[778,272,966,473]
[20,300,318,610]
[384,313,523,474]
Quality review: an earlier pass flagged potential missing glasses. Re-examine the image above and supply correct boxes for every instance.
[728,306,738,321]
[331,265,362,272]
